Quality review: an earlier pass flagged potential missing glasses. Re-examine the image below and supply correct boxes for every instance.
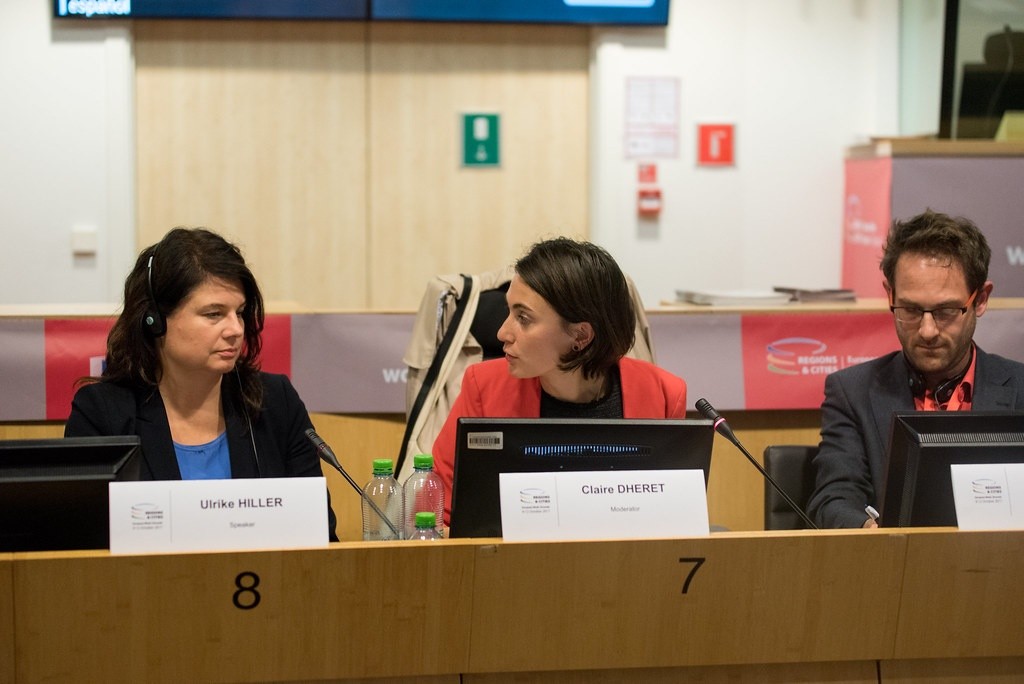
[887,288,977,324]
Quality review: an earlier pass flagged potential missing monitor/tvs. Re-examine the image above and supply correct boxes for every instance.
[0,436,150,552]
[875,409,1024,527]
[449,417,714,537]
[955,62,1024,140]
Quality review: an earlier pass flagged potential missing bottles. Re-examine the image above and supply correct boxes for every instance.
[360,459,405,541]
[403,455,444,536]
[408,512,443,541]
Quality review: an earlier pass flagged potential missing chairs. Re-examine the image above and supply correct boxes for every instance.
[764,443,817,531]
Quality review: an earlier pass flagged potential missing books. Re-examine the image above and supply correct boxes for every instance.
[673,280,856,307]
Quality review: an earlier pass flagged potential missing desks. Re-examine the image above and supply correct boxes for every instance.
[0,296,1024,540]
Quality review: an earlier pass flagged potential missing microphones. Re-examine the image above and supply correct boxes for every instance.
[304,429,403,538]
[694,399,817,530]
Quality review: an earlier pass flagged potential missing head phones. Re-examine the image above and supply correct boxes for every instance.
[141,244,168,338]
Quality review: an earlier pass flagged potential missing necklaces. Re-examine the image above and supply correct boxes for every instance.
[597,373,604,403]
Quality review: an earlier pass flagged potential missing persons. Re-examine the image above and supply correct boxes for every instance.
[64,225,342,543]
[410,233,690,542]
[800,207,1024,532]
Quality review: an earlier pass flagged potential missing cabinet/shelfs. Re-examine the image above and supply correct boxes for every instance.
[841,140,1024,295]
